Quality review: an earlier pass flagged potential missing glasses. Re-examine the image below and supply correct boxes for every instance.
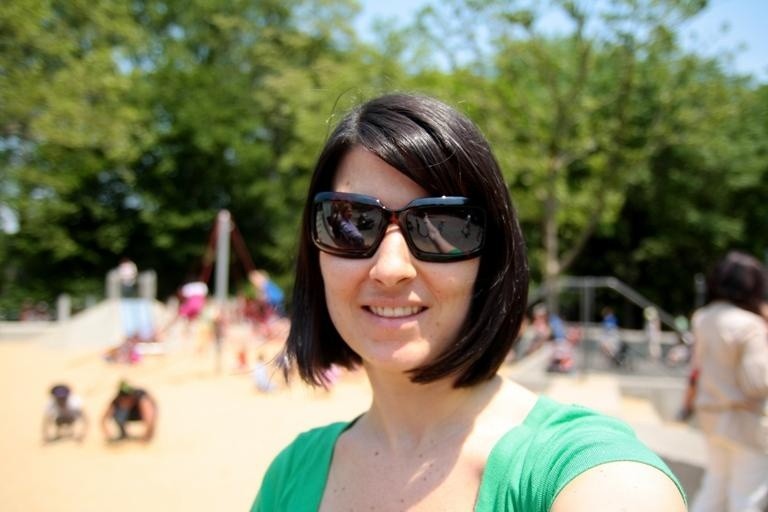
[304,190,487,264]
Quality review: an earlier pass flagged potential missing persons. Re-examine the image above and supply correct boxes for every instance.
[533,301,575,374]
[100,380,157,444]
[243,94,690,512]
[39,384,92,442]
[681,248,767,511]
